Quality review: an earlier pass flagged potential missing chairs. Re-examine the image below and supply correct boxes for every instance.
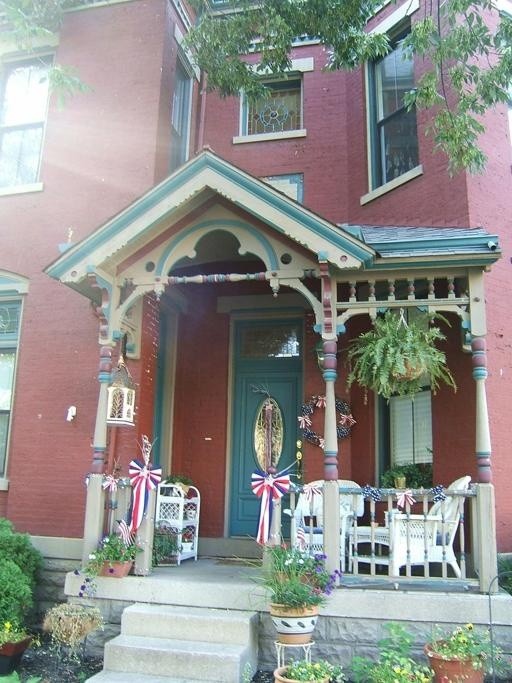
[283,475,472,578]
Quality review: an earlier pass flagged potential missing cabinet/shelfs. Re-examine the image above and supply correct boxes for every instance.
[155,479,201,566]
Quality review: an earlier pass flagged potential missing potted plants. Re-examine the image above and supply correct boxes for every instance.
[381,447,434,490]
[165,475,194,497]
[343,307,460,408]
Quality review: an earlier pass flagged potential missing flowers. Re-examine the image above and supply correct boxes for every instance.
[181,528,193,542]
[232,531,344,624]
[285,622,512,683]
[74,530,145,599]
[0,620,31,651]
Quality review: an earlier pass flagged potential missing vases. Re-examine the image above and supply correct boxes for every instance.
[0,635,32,676]
[274,666,330,683]
[269,603,320,645]
[185,510,196,521]
[182,543,193,552]
[98,560,135,578]
